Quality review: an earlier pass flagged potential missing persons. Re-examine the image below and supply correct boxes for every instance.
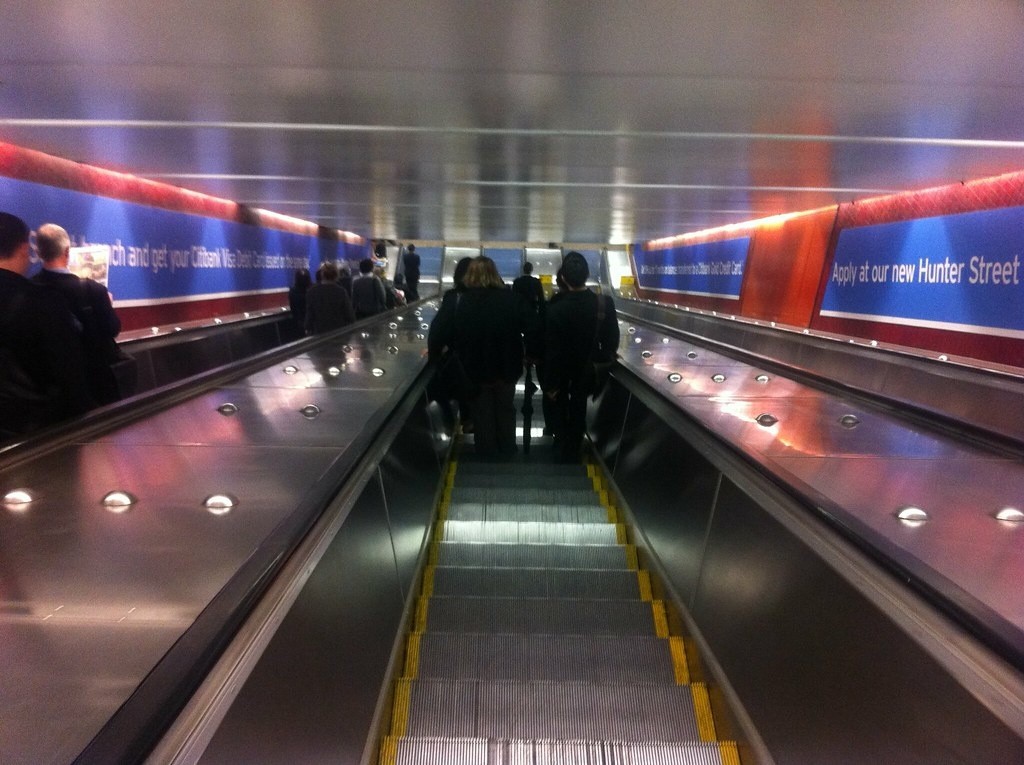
[287,241,420,338]
[425,253,620,462]
[0,212,121,449]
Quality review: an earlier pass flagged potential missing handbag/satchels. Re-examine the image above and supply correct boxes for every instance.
[542,366,580,406]
[101,357,139,404]
